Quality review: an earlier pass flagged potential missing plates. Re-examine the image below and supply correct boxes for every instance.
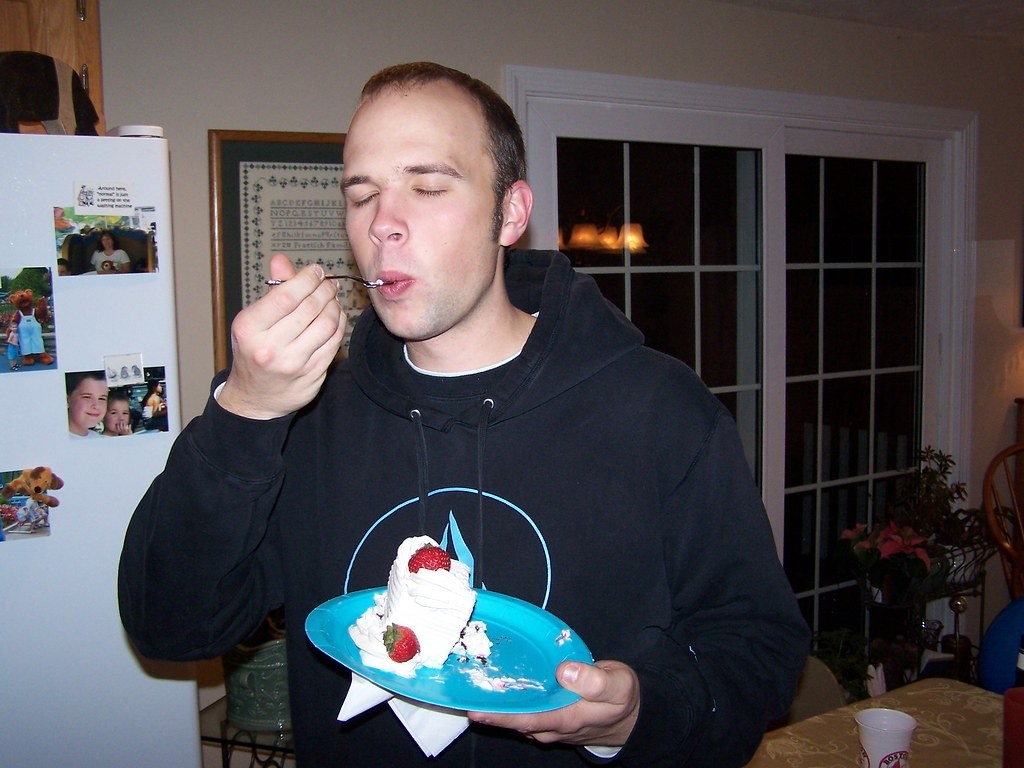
[305,586,594,713]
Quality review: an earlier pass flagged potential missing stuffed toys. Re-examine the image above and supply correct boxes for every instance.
[2,466,64,507]
[7,289,54,365]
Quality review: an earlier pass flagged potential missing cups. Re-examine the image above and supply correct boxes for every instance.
[853,707,918,768]
[1003,688,1024,768]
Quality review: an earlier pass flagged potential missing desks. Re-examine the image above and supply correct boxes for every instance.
[745,675,1005,768]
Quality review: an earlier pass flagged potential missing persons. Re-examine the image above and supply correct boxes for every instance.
[90,231,131,274]
[57,258,72,276]
[117,61,812,768]
[66,372,168,438]
[7,322,21,371]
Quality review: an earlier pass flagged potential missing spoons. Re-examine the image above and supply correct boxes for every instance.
[265,273,392,288]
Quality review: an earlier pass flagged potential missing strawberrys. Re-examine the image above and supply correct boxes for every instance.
[383,624,421,662]
[408,543,452,571]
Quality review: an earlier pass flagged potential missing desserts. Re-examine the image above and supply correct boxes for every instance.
[346,535,494,678]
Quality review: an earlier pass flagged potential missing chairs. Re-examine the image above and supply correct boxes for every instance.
[982,441,1024,601]
[787,655,847,726]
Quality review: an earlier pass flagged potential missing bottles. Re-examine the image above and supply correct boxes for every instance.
[1015,633,1024,687]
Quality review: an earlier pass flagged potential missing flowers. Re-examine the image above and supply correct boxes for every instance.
[836,444,981,576]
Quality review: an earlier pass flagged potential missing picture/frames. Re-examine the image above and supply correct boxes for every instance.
[208,127,371,376]
